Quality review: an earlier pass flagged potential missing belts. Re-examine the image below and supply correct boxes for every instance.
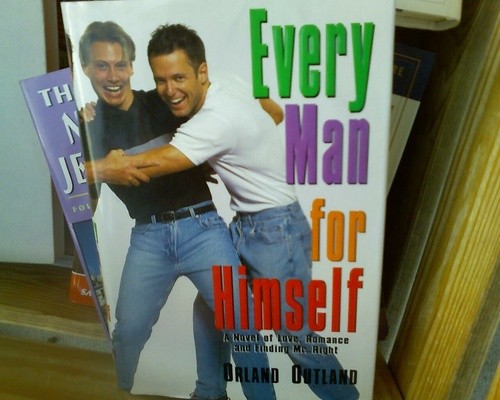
[135,202,217,226]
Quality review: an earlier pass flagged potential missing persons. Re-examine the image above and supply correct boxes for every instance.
[79,20,277,400]
[79,23,361,400]
